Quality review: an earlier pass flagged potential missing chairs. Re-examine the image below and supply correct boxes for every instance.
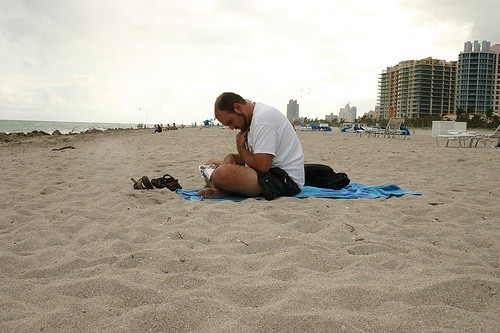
[384,117,407,140]
[469,124,500,149]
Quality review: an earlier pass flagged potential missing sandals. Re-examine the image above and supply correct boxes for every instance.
[151,174,182,192]
[131,176,153,189]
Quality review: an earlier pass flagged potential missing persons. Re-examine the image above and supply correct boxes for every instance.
[154,124,162,133]
[165,123,170,130]
[198,92,305,201]
[173,123,176,130]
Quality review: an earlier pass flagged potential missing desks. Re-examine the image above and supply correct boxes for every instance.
[436,135,477,147]
